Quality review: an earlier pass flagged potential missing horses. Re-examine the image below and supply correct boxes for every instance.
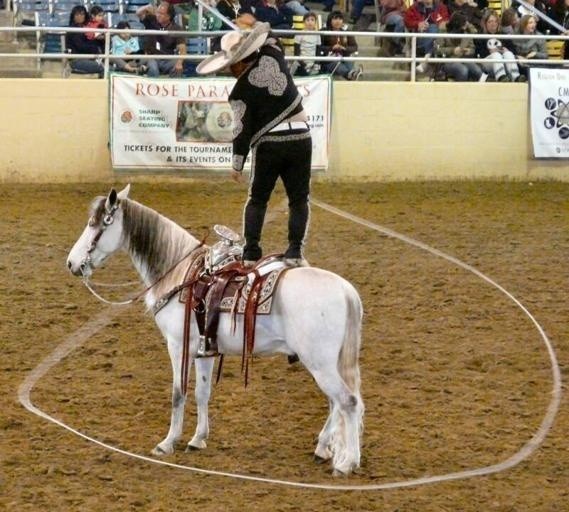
[66,183,366,478]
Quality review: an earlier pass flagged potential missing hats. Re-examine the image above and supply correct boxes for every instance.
[196,22,270,74]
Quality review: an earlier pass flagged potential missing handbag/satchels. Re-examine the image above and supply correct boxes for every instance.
[377,38,396,56]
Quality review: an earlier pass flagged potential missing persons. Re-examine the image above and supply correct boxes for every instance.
[195,21,312,266]
[63,0,568,81]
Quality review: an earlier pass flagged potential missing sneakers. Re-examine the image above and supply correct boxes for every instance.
[417,64,428,73]
[136,65,147,74]
[243,251,260,267]
[351,65,363,80]
[515,75,526,82]
[497,75,510,82]
[285,252,301,267]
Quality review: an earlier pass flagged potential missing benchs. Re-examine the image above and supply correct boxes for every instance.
[12,1,208,78]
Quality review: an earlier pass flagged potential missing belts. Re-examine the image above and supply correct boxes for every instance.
[269,121,308,132]
